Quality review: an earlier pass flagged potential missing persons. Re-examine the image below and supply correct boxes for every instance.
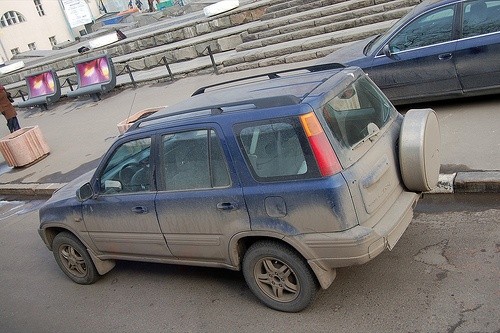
[148,0,160,12]
[135,0,143,11]
[0,83,20,133]
[128,0,133,10]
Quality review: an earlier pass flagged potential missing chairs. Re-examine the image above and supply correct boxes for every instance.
[163,141,216,190]
[18,67,61,111]
[469,1,487,36]
[68,54,116,102]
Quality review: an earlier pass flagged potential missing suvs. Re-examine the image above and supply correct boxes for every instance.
[38,62,441,312]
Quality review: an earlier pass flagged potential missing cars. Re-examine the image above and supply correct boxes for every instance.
[313,0,500,111]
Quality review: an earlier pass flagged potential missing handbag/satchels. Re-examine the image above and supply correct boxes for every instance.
[7,94,14,103]
